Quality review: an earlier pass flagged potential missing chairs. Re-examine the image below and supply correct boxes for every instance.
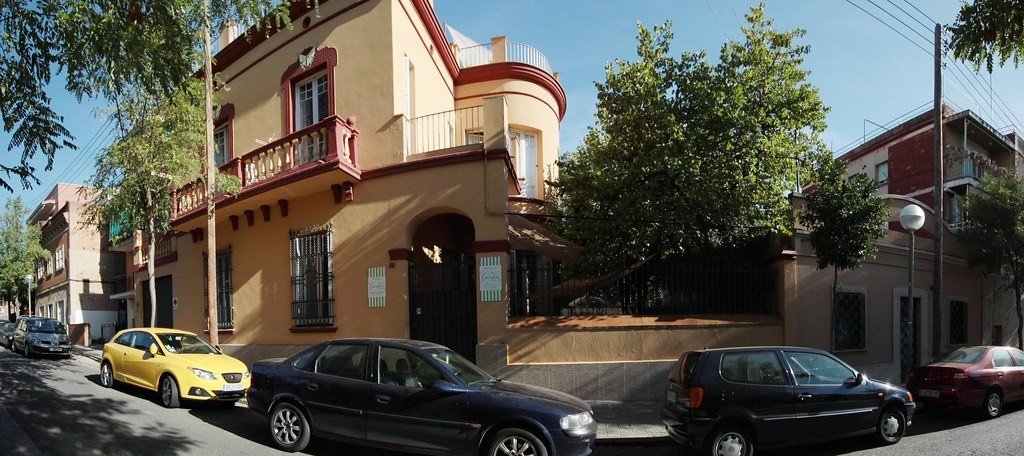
[41,323,48,331]
[380,359,394,386]
[395,358,415,387]
[759,362,793,384]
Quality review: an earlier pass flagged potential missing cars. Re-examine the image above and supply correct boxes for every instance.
[0,320,16,349]
[246,337,599,456]
[905,345,1024,419]
[567,294,613,309]
[99,327,251,408]
[659,345,916,456]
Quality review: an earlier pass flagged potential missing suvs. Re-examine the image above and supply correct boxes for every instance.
[10,315,74,359]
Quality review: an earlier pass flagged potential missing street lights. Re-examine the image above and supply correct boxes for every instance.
[899,202,927,387]
[24,274,34,317]
